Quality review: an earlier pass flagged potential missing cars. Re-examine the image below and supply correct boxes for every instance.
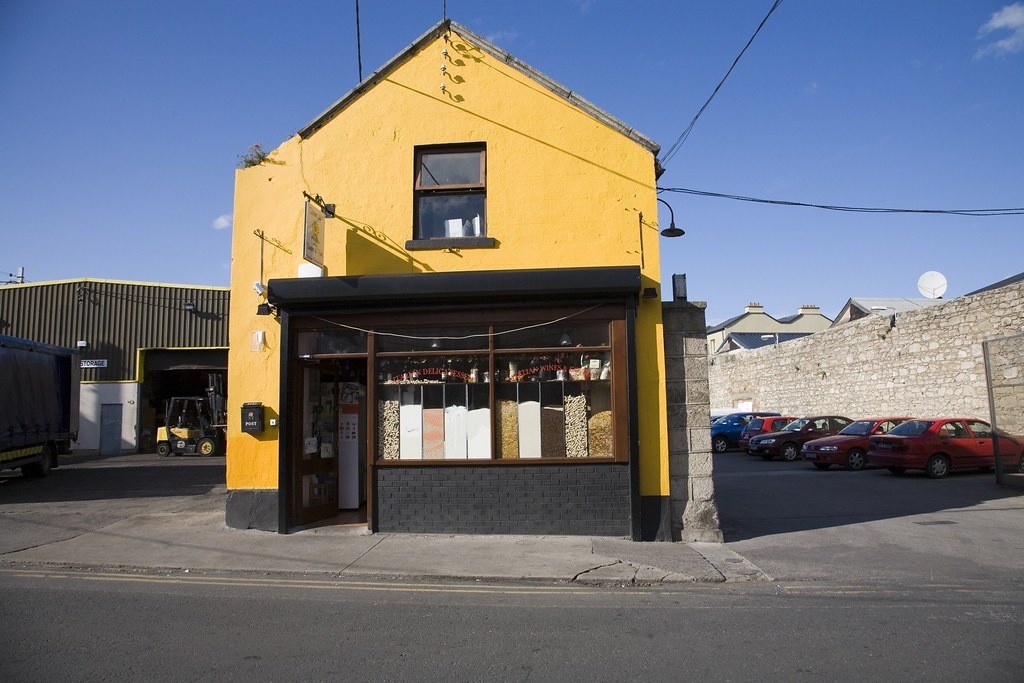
[711,412,781,453]
[868,418,1024,480]
[748,415,855,463]
[801,415,918,471]
[738,415,800,454]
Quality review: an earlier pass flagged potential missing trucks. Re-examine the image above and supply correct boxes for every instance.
[0,333,80,477]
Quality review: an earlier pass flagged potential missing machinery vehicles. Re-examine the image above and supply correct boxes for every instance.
[156,373,227,458]
